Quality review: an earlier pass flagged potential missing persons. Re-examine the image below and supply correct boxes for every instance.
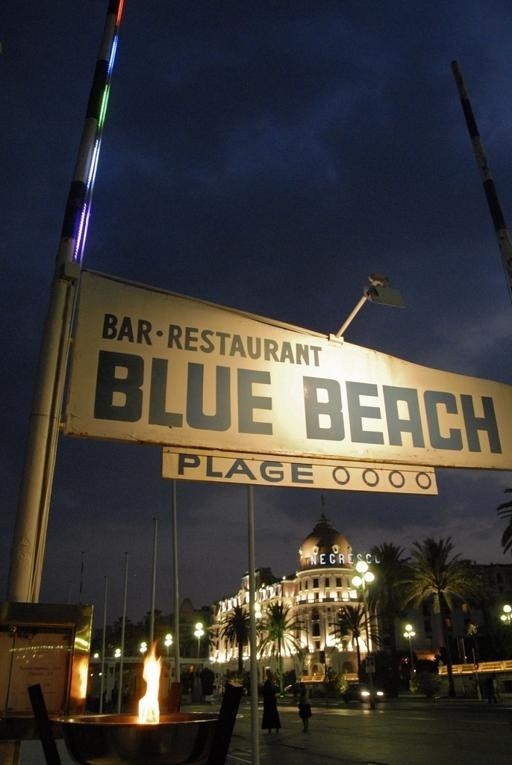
[297,682,313,732]
[484,676,497,704]
[261,678,282,736]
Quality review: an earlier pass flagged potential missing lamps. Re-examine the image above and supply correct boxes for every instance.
[327,272,406,344]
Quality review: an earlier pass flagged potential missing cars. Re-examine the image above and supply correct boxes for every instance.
[343,685,384,704]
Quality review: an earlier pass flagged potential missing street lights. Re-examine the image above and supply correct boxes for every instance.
[403,625,415,669]
[352,562,376,709]
[193,622,205,658]
[164,633,174,658]
